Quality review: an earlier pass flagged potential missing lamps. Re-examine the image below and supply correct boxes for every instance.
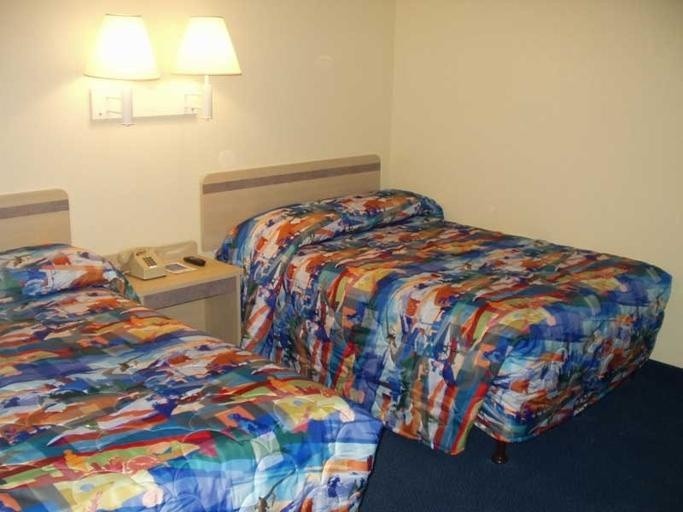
[85,11,163,127]
[170,15,241,119]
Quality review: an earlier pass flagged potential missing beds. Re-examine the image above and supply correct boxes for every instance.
[0,190,385,512]
[199,154,673,464]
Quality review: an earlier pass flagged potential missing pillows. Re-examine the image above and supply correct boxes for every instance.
[0,242,141,306]
[214,189,444,352]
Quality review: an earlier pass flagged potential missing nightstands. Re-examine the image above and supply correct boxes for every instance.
[106,240,245,349]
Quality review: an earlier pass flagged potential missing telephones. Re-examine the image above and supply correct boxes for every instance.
[118,247,167,279]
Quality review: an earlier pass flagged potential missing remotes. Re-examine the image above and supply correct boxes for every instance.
[183,256,205,266]
